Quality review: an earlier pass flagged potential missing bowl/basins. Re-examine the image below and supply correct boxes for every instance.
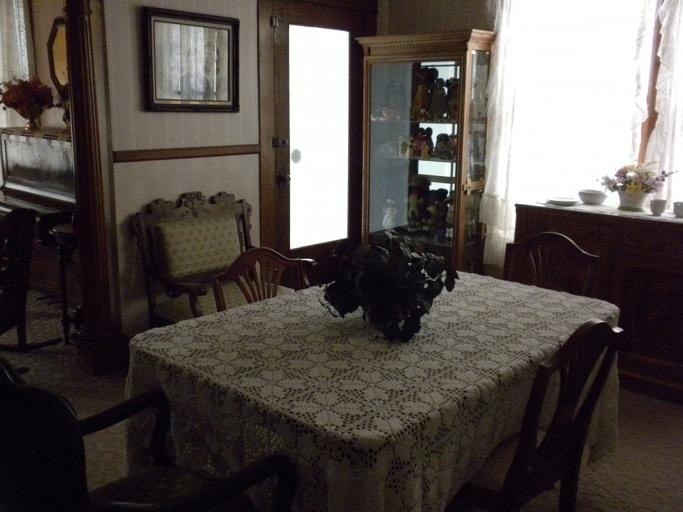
[577,189,608,206]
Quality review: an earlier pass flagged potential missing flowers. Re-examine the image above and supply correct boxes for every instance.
[0,75,54,119]
[594,164,679,194]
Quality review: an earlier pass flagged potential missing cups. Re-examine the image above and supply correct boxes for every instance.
[650,199,667,217]
[673,201,683,217]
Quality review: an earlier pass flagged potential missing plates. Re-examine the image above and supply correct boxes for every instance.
[548,199,578,206]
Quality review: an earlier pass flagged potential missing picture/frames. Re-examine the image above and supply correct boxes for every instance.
[134,4,241,114]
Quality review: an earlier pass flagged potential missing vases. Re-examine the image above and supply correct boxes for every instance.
[25,115,43,132]
[615,184,650,212]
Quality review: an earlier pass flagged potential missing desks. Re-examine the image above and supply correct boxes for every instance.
[125,268,621,512]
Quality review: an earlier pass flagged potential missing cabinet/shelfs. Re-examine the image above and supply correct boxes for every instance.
[511,200,683,403]
[354,28,497,274]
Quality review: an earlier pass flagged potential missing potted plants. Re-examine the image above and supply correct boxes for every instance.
[319,228,458,338]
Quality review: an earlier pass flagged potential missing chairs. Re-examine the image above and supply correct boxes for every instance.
[524,231,600,299]
[0,201,37,346]
[458,317,625,512]
[202,246,316,312]
[123,189,296,327]
[0,344,295,512]
